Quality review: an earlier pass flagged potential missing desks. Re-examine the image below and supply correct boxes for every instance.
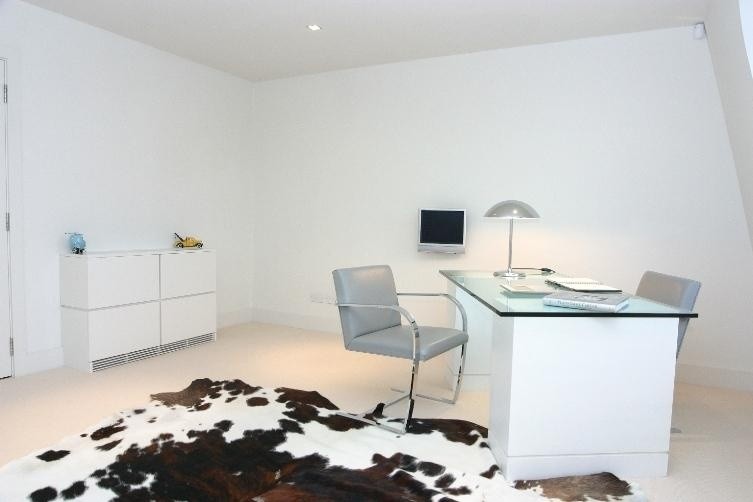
[439,269,699,482]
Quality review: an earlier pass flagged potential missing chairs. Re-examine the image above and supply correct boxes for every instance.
[333,265,470,434]
[636,272,701,361]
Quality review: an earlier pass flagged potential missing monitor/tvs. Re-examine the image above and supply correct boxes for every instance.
[417,208,466,254]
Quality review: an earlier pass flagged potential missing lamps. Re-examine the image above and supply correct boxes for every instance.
[484,200,541,277]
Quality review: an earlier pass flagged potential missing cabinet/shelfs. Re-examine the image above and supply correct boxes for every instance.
[58,250,216,375]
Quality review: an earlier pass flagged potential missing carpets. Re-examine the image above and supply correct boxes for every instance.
[2,378,646,501]
